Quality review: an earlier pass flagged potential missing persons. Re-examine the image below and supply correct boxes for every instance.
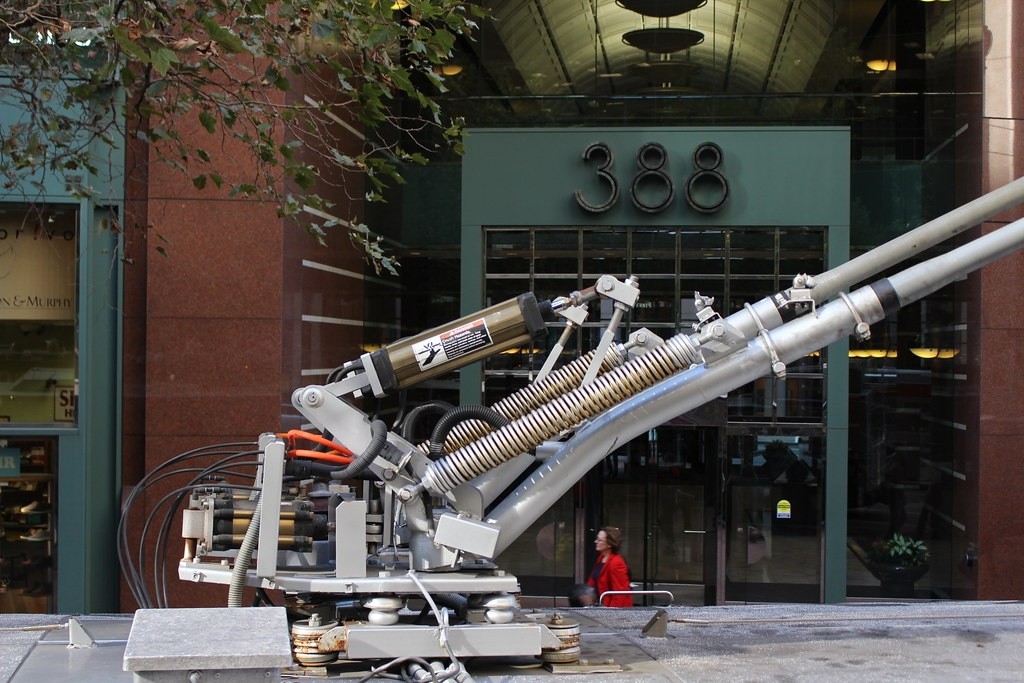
[587,525,633,607]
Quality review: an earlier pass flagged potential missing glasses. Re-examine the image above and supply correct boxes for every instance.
[596,537,606,543]
